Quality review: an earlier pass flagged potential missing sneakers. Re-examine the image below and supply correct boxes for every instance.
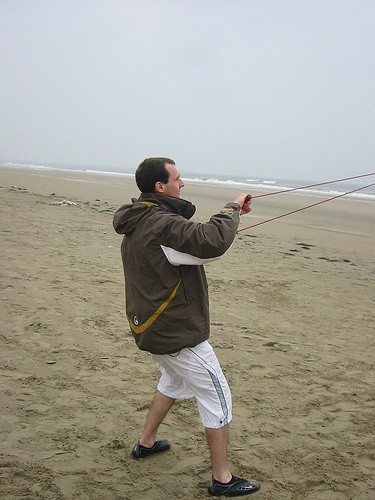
[131,439,171,459]
[208,473,261,497]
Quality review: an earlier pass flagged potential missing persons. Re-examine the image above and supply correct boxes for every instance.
[111,158,262,496]
